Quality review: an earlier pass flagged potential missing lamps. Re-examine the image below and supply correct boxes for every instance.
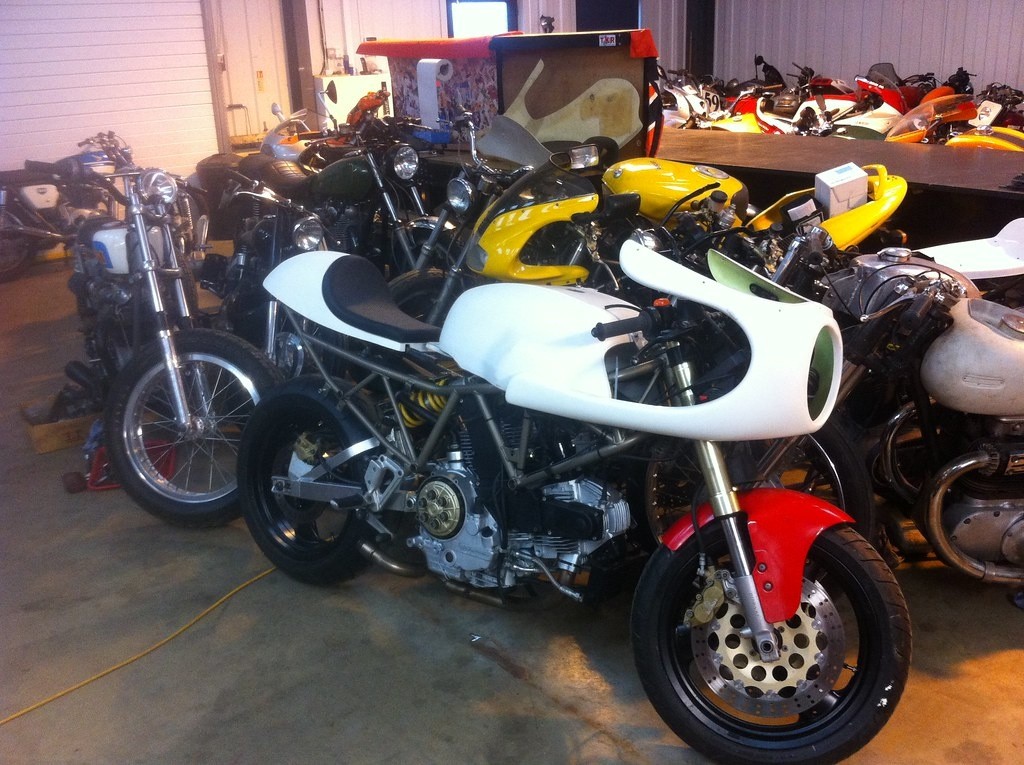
[540,14,555,32]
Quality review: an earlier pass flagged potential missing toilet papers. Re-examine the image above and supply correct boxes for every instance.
[417,57,455,129]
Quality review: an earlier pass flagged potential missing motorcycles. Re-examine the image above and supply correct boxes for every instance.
[180,14,1024,619]
[234,190,916,765]
[0,129,173,285]
[63,131,284,531]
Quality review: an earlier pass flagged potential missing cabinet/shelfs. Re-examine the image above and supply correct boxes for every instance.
[312,73,394,132]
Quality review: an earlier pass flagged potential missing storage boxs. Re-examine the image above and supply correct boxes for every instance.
[814,162,868,218]
